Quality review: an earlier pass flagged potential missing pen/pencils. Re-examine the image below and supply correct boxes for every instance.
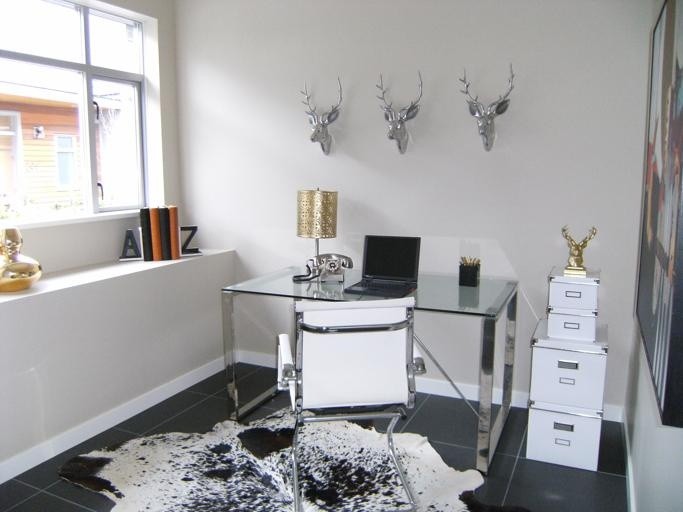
[459,255,481,267]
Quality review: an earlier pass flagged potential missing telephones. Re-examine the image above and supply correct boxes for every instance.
[313,254,353,282]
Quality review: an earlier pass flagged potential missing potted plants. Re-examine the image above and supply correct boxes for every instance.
[457,256,480,287]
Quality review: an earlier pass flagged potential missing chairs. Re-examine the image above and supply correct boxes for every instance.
[274,294,424,512]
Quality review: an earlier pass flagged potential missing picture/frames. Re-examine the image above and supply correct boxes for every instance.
[631,0,680,431]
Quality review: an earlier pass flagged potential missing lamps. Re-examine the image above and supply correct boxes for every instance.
[294,187,339,273]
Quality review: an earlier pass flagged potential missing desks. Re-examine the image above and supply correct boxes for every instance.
[221,264,518,475]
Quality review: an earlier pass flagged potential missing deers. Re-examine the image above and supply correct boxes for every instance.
[457,62,515,152]
[375,70,423,154]
[300,76,343,155]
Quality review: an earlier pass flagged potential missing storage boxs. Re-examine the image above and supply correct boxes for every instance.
[523,408,601,475]
[548,265,601,316]
[528,316,607,416]
[544,307,598,344]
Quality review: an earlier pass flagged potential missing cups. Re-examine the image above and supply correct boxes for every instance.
[457,285,479,308]
[458,263,480,287]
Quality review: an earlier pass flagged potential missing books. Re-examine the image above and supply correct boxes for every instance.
[140,206,179,261]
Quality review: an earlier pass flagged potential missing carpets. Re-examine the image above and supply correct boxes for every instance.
[57,402,530,512]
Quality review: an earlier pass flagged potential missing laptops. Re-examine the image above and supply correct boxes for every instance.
[344,235,421,299]
